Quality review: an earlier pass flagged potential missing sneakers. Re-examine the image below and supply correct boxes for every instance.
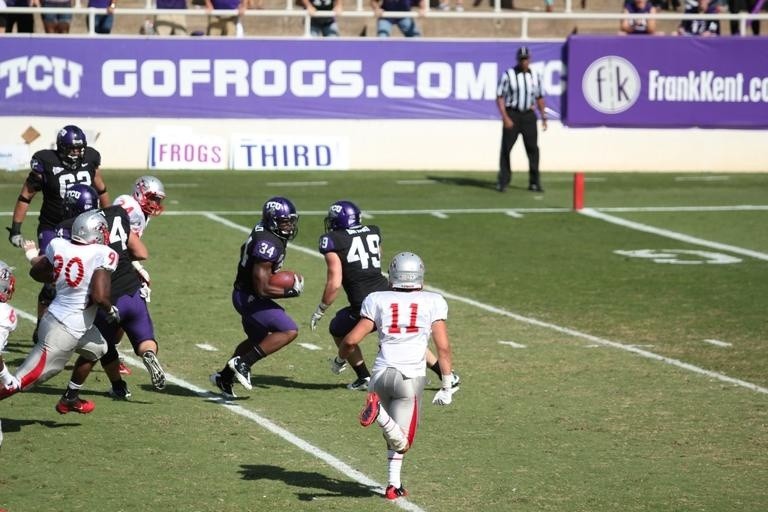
[495,182,505,191]
[359,391,382,426]
[351,373,370,391]
[384,483,409,500]
[449,372,462,390]
[210,371,236,398]
[529,183,540,191]
[111,379,133,399]
[57,398,95,413]
[227,355,253,390]
[143,350,165,391]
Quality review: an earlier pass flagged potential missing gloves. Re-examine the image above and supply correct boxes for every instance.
[9,233,23,246]
[431,382,453,406]
[310,303,329,331]
[333,357,348,375]
[293,274,305,294]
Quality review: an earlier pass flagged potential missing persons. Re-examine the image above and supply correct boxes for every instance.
[299,0,464,37]
[328,249,461,503]
[0,0,114,33]
[203,196,304,402]
[6,126,110,344]
[622,0,768,36]
[0,175,168,414]
[489,44,549,196]
[310,198,459,391]
[149,0,245,35]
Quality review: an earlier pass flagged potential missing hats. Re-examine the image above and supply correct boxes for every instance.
[516,47,529,60]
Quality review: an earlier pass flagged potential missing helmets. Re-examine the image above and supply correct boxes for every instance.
[263,196,298,240]
[57,126,86,168]
[71,211,110,246]
[134,175,167,216]
[0,260,18,303]
[388,252,424,292]
[323,201,363,233]
[64,184,100,216]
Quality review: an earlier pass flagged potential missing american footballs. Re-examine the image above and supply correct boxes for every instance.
[268,271,302,290]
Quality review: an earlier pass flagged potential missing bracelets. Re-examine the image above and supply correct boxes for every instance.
[283,288,297,300]
